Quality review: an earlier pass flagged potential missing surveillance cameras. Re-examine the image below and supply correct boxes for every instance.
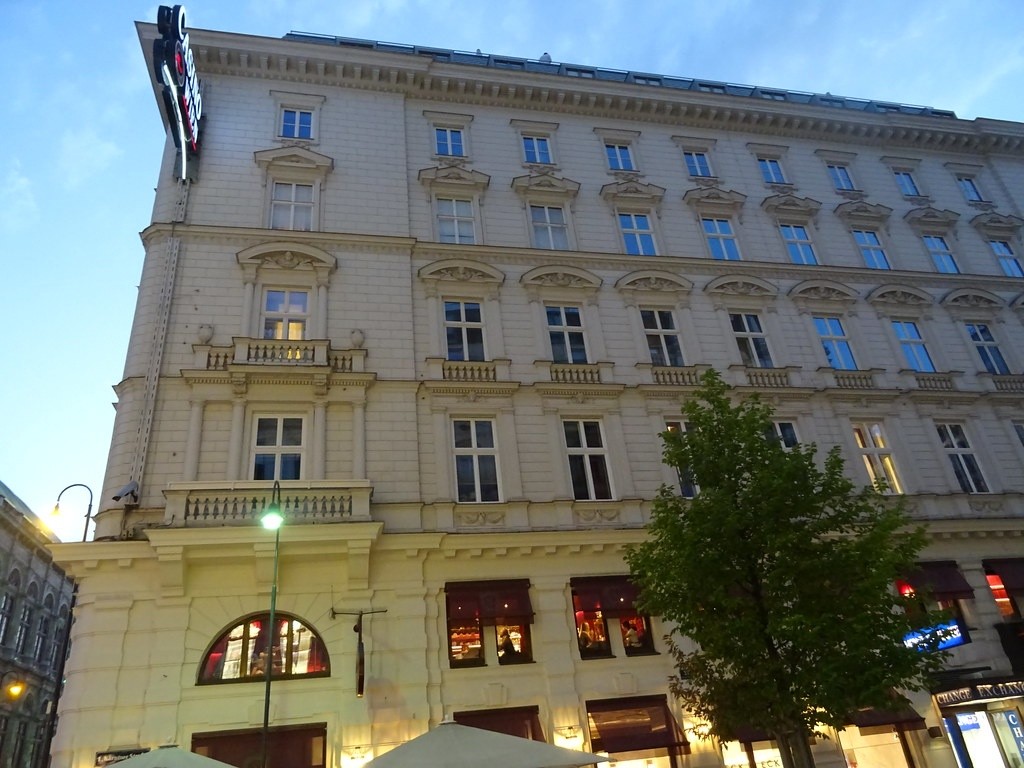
[112,482,138,502]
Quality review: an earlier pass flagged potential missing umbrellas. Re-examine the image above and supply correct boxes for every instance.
[361,715,612,768]
[102,738,238,768]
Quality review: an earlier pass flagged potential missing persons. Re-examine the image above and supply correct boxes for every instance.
[498,629,516,658]
[579,622,595,650]
[461,643,469,657]
[622,621,639,647]
[250,654,258,675]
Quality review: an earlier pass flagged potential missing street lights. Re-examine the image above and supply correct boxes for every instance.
[41,484,93,768]
[259,480,284,768]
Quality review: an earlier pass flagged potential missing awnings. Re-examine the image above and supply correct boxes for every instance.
[905,562,976,600]
[456,716,546,743]
[839,685,927,736]
[586,698,691,762]
[446,590,534,628]
[718,691,817,751]
[576,581,664,619]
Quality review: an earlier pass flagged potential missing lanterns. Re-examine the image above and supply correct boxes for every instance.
[902,585,915,598]
